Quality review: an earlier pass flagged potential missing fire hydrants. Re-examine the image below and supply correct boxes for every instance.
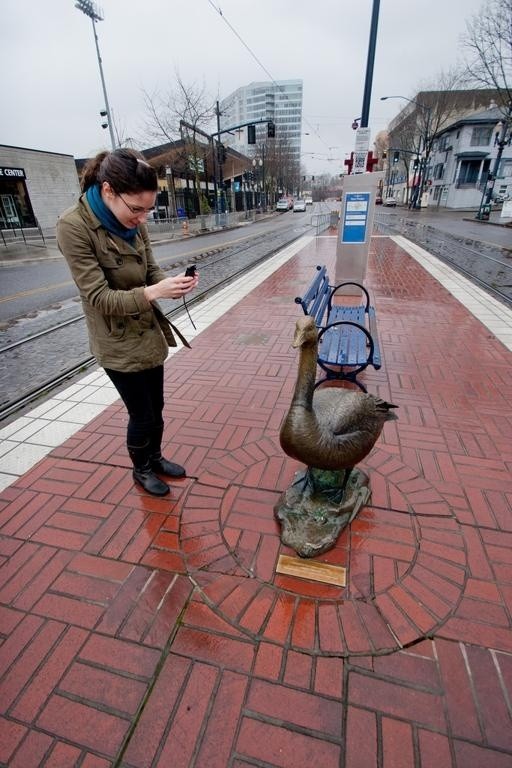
[182,221,189,235]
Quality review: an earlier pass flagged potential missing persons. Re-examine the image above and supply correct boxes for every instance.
[54,146,200,498]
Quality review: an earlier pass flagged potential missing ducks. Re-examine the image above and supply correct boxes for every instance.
[279,316,399,507]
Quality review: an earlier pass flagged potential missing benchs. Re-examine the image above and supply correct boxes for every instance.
[294,262,382,394]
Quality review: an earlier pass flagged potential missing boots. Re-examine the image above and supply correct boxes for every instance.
[127,441,170,496]
[149,422,185,477]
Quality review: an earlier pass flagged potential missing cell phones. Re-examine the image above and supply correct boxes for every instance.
[185,264,197,277]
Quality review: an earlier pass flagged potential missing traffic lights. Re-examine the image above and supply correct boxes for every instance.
[268,123,274,137]
[393,152,399,162]
[413,160,419,170]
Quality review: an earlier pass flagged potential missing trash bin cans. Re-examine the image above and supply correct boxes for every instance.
[178,208,186,223]
[480,204,491,220]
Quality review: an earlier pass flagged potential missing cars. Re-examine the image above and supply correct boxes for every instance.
[277,196,313,212]
[383,197,396,207]
[376,197,382,203]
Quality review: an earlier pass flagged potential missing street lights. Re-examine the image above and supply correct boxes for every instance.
[380,96,430,206]
[280,133,309,176]
[252,158,263,214]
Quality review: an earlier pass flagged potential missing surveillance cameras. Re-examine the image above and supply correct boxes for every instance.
[352,123,358,130]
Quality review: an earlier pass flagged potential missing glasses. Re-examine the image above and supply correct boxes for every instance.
[117,192,156,215]
